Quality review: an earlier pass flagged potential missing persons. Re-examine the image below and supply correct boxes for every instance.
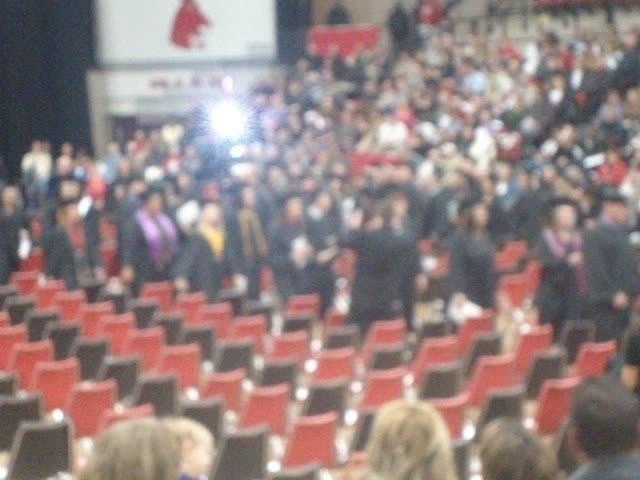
[163,416,215,479]
[2,0,638,396]
[478,416,547,480]
[77,419,180,479]
[567,375,640,480]
[366,399,457,480]
